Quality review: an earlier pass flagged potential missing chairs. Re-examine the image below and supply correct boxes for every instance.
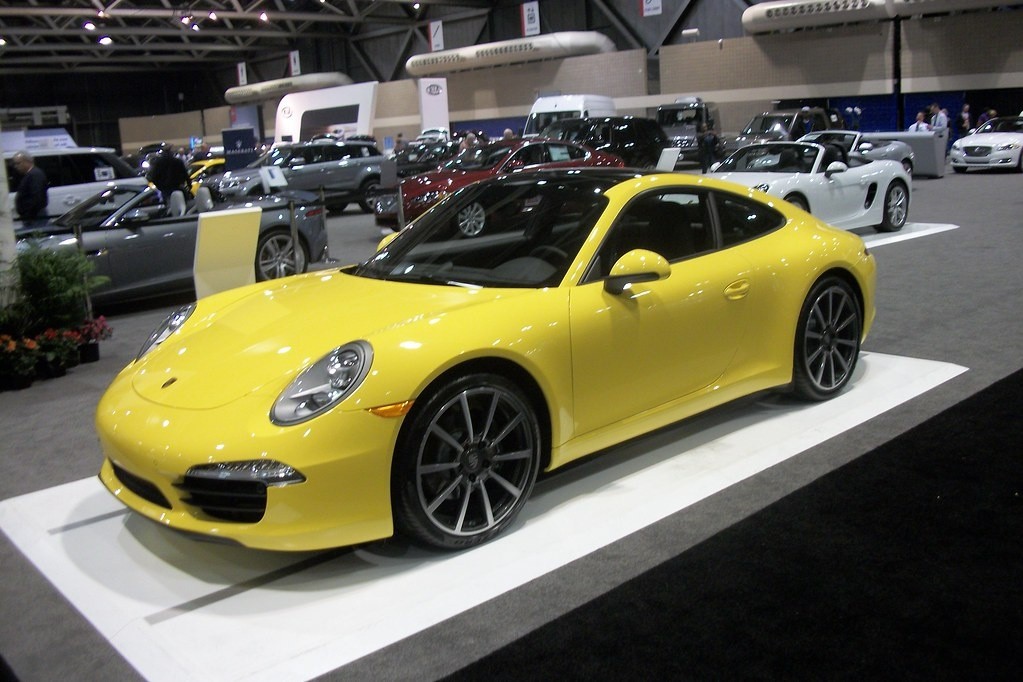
[807,144,838,174]
[649,201,697,259]
[779,149,798,172]
[166,189,187,219]
[188,187,213,216]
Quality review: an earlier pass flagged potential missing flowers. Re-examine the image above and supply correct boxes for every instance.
[0,335,39,374]
[82,316,113,343]
[36,330,81,365]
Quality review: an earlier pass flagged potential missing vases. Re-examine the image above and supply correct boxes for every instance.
[81,342,101,364]
[2,373,32,391]
[36,356,66,379]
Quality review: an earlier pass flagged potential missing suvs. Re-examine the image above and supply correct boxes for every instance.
[0,146,165,236]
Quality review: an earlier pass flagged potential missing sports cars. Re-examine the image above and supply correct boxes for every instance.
[17,181,327,312]
[747,128,917,184]
[949,115,1023,174]
[94,165,878,553]
[657,140,914,234]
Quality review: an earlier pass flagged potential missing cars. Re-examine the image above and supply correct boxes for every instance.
[114,96,852,251]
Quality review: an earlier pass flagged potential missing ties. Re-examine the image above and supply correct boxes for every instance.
[933,113,938,126]
[915,122,920,132]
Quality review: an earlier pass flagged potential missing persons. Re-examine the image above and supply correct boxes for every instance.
[695,121,721,174]
[908,112,930,131]
[926,104,948,132]
[395,132,408,153]
[140,144,214,169]
[956,104,973,139]
[448,128,524,155]
[394,139,406,153]
[12,151,49,228]
[253,141,271,154]
[146,143,192,204]
[941,107,954,140]
[793,106,821,142]
[976,110,999,133]
